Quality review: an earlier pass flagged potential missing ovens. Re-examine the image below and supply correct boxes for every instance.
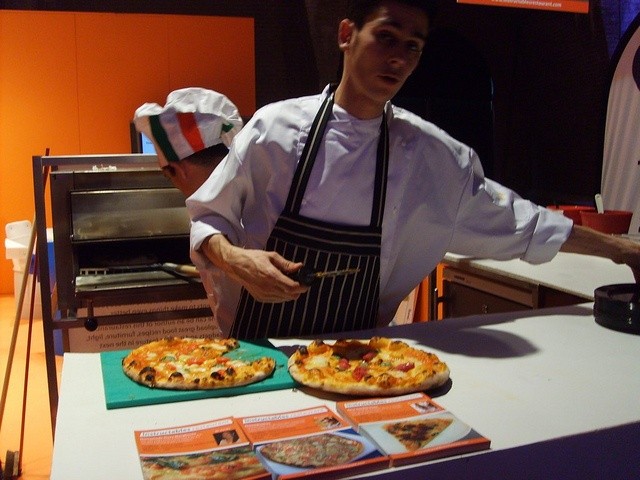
[52,161,208,332]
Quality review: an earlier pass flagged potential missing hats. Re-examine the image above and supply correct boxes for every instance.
[134,86,244,170]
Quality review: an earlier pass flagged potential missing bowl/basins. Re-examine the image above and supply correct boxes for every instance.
[545,204,597,225]
[579,208,633,234]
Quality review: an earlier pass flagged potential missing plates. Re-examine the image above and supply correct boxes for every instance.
[255,429,374,475]
[361,410,472,455]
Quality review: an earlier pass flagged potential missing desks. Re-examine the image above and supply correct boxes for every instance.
[48,298,640,480]
[443,241,636,301]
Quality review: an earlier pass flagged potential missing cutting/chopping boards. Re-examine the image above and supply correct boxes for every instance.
[101,337,302,410]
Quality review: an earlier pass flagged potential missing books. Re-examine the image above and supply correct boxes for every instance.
[337,392,491,466]
[134,417,272,479]
[234,406,389,480]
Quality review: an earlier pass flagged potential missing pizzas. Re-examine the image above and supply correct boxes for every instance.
[123,338,275,388]
[261,434,362,467]
[288,338,449,395]
[142,448,266,480]
[384,419,450,450]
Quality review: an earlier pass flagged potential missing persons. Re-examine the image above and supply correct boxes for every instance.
[132,87,244,338]
[187,0,639,344]
